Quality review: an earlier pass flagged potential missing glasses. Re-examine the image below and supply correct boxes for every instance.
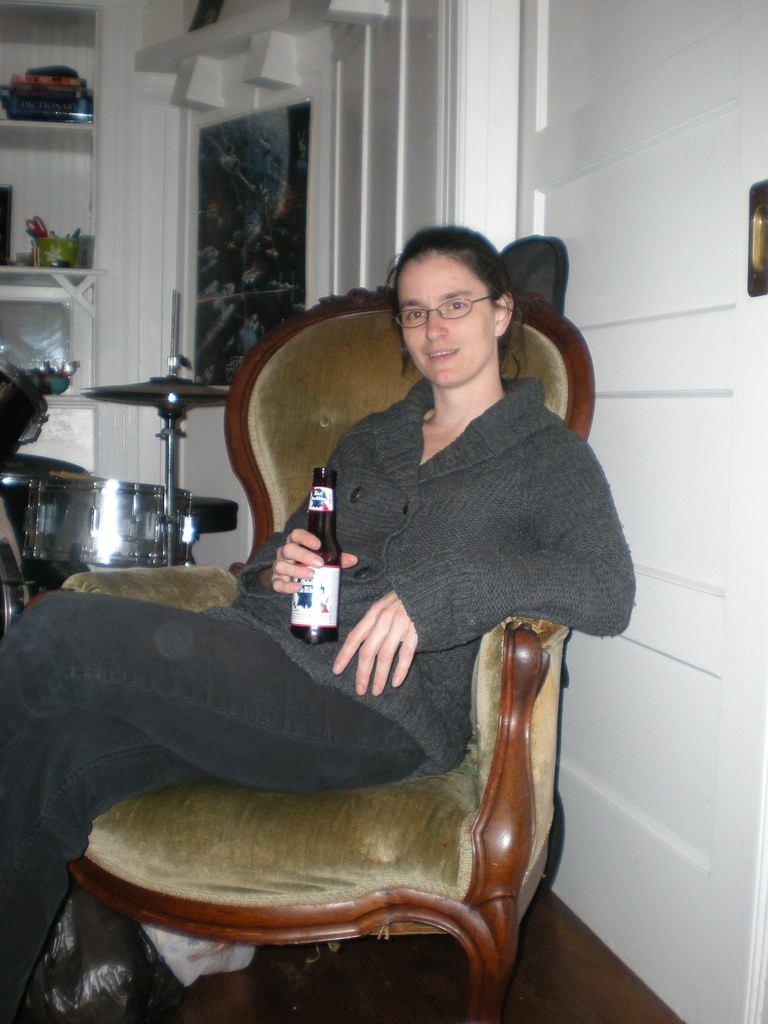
[391,295,497,327]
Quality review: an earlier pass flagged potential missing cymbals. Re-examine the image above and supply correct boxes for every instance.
[80,376,229,409]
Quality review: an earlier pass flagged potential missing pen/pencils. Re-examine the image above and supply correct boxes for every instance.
[25,229,35,237]
[50,228,80,239]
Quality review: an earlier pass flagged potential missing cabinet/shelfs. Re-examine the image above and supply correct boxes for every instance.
[0,0,102,478]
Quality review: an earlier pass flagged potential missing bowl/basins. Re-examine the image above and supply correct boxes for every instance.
[34,238,79,267]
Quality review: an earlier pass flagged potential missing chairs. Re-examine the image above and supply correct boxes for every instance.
[21,286,596,1024]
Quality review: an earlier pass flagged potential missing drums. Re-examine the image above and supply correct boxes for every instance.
[22,478,193,568]
[0,355,48,465]
[0,542,91,638]
[1,453,89,551]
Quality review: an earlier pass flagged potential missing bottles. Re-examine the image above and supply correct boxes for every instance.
[290,467,343,644]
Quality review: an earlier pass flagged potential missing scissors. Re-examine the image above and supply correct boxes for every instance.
[27,216,48,237]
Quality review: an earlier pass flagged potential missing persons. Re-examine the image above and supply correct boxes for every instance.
[0,225,636,1024]
